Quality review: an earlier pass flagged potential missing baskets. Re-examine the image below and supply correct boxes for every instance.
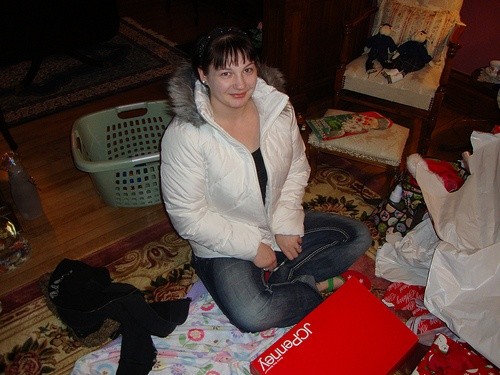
[71,100,175,208]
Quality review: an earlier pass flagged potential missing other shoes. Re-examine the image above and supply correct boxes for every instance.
[316,274,345,292]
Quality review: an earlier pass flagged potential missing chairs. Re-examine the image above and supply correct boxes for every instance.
[334,0,464,159]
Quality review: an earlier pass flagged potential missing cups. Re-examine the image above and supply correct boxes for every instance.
[490,61,500,72]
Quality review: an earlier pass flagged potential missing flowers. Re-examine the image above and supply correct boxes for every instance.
[249,21,263,48]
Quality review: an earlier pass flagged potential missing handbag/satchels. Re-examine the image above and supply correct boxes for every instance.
[366,157,469,244]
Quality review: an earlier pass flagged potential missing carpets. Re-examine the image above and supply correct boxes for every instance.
[0,163,430,375]
[0,17,194,128]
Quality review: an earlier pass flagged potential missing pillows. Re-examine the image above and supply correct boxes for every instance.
[364,0,466,72]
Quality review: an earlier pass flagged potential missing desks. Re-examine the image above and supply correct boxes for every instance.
[471,66,500,118]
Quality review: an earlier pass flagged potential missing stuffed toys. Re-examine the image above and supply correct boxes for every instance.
[381,31,435,84]
[362,23,396,73]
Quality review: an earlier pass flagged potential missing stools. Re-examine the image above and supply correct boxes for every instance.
[307,108,411,197]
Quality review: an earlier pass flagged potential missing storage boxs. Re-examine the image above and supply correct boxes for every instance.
[411,333,500,375]
[250,276,421,375]
[71,99,176,208]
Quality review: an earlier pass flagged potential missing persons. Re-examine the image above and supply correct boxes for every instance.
[158,24,371,335]
